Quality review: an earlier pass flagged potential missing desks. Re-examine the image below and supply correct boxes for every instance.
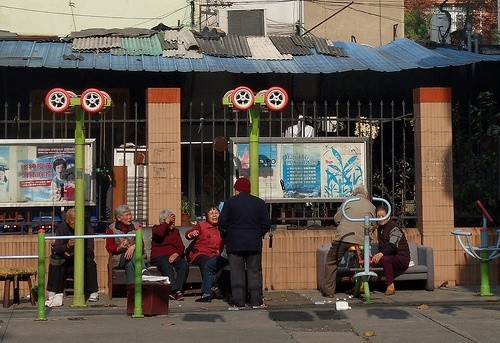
[0,266,36,309]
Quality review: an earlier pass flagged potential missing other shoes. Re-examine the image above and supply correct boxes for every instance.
[169,293,177,299]
[327,294,334,298]
[88,293,99,302]
[177,292,184,301]
[252,305,260,308]
[385,289,395,295]
[230,302,245,309]
[194,297,211,302]
[346,287,364,294]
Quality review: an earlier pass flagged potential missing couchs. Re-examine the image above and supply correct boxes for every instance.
[316,241,435,291]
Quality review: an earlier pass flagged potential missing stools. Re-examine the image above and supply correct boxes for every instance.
[64,265,74,296]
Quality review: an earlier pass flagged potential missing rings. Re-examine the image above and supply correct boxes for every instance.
[376,259,378,260]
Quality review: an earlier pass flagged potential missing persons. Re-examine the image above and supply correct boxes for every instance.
[321,185,376,298]
[218,178,271,308]
[185,206,248,302]
[106,205,152,283]
[150,209,189,301]
[51,158,75,200]
[45,209,99,306]
[372,206,410,295]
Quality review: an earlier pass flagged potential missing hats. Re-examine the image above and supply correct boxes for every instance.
[233,178,251,193]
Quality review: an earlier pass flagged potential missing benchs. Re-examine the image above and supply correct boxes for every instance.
[106,226,203,300]
